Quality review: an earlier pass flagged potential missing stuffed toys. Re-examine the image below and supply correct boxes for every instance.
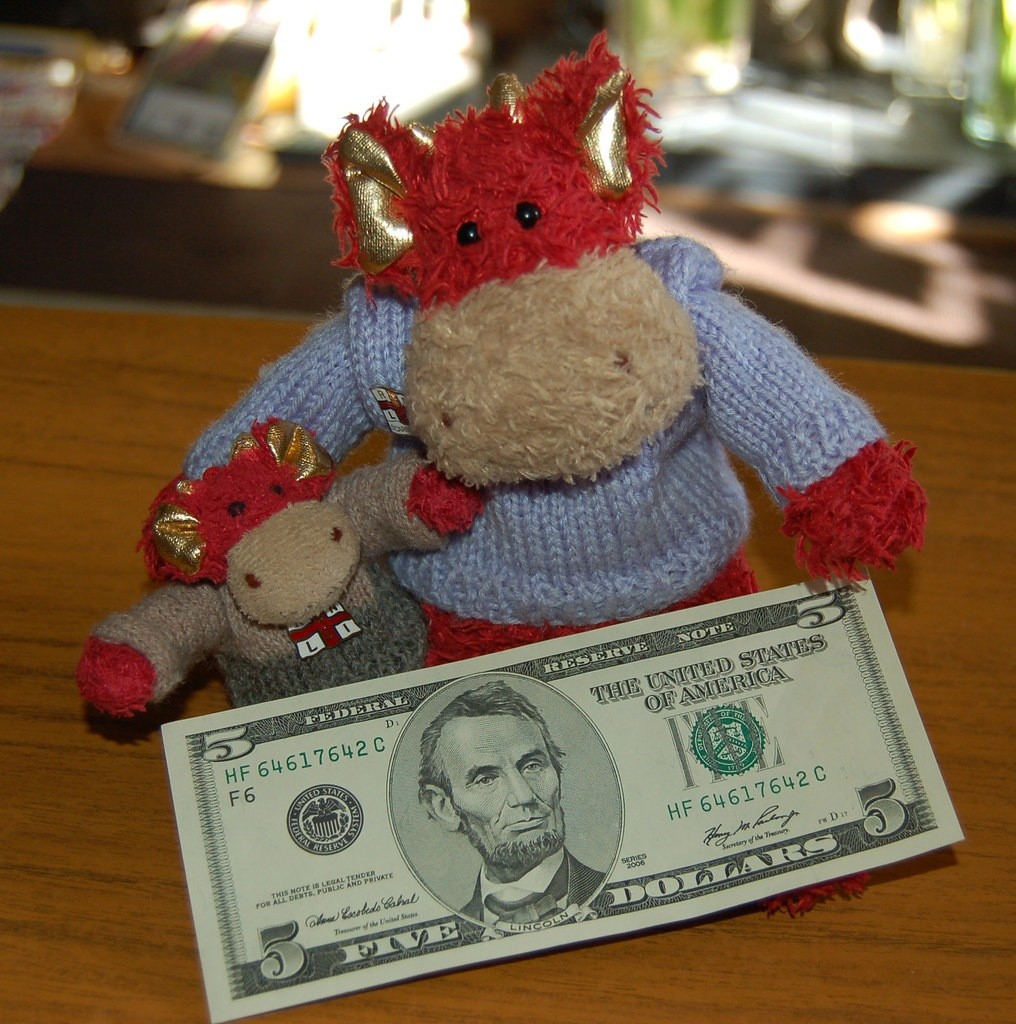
[135,29,929,666]
[79,410,484,718]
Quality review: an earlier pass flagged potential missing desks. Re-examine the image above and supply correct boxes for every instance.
[0,302,1016,1024]
